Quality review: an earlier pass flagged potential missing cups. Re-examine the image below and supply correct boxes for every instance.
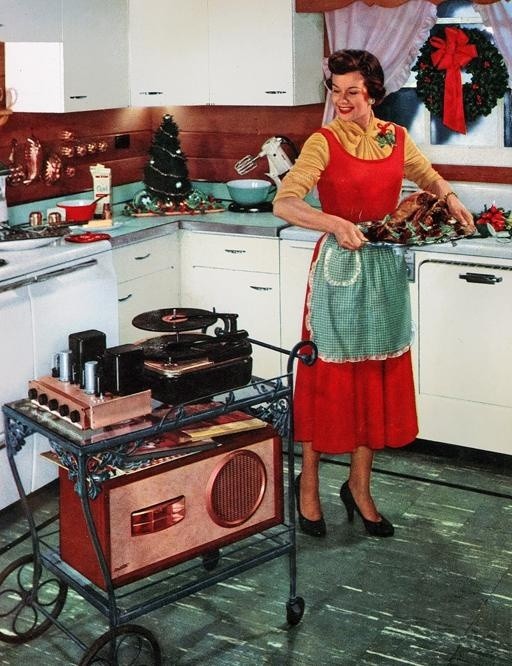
[49,213,61,225]
[84,360,98,396]
[59,349,73,381]
[29,212,43,226]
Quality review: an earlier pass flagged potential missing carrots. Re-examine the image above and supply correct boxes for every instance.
[476,206,506,231]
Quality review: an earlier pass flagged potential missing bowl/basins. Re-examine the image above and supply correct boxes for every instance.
[56,200,96,224]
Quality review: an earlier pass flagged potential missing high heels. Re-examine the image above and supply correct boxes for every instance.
[293,474,325,538]
[340,478,393,537]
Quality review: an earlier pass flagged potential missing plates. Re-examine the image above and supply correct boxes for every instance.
[354,215,476,247]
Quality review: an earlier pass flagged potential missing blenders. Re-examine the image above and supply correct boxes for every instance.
[226,135,301,214]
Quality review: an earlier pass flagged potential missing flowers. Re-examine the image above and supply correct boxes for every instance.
[470,202,512,238]
[412,23,511,135]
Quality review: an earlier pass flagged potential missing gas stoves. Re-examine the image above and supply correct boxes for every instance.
[0,225,119,284]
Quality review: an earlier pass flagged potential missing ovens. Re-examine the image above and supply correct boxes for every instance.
[0,248,144,510]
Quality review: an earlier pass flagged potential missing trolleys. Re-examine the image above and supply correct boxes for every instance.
[2,304,319,666]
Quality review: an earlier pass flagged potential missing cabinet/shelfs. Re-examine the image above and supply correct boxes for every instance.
[279,239,317,431]
[0,0,326,113]
[112,230,180,346]
[417,250,512,456]
[179,228,281,412]
